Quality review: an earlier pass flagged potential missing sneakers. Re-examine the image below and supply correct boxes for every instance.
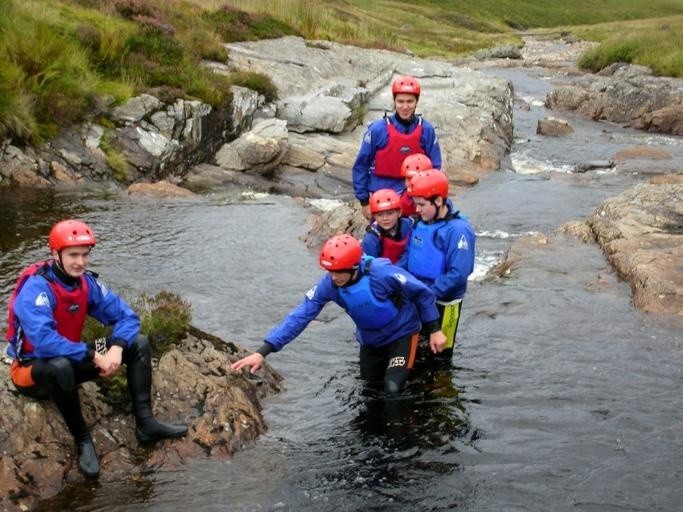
[76,433,100,475]
[134,422,188,445]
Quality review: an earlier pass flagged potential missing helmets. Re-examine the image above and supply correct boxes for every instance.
[401,153,432,178]
[392,76,421,94]
[406,168,449,199]
[370,188,401,213]
[319,234,362,270]
[49,220,96,250]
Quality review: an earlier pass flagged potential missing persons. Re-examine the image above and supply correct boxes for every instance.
[357,188,414,264]
[393,168,474,372]
[231,233,447,397]
[352,75,442,223]
[395,153,453,216]
[6,220,188,478]
